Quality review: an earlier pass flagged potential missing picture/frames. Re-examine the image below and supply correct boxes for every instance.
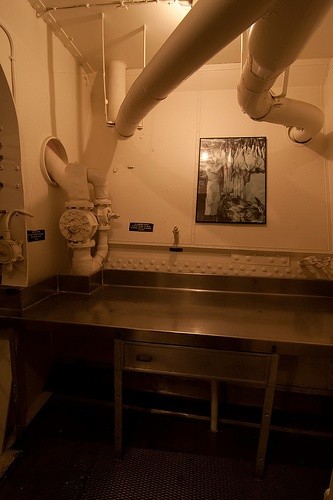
[195,136,268,226]
